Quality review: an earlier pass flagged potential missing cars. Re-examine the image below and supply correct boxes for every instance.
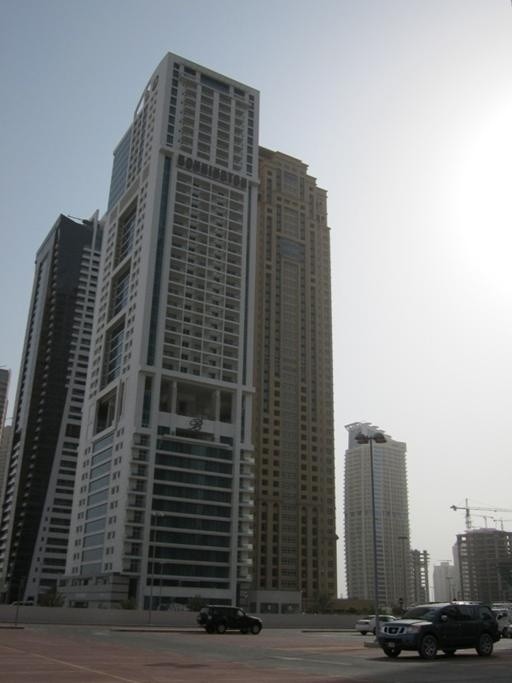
[356,615,398,634]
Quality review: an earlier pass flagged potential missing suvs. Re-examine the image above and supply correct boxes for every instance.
[374,602,500,656]
[196,604,263,634]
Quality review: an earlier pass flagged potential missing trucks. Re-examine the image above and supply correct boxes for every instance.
[489,601,512,638]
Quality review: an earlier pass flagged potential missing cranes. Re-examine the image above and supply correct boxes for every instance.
[450,498,512,531]
